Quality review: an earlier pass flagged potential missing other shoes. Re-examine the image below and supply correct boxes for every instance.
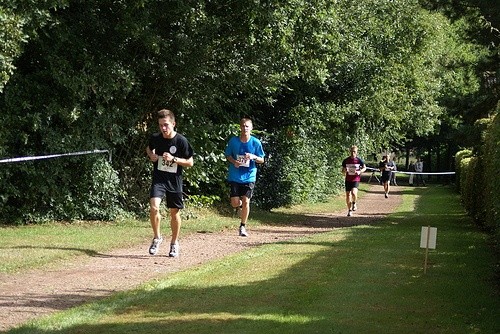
[352,202,357,211]
[239,226,247,236]
[347,210,351,216]
[385,192,389,199]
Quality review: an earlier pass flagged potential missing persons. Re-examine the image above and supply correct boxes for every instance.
[225,118,266,237]
[342,146,367,216]
[380,152,398,199]
[415,157,426,186]
[146,109,194,257]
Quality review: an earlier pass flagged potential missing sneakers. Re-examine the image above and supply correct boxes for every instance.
[169,241,181,257]
[149,237,163,255]
